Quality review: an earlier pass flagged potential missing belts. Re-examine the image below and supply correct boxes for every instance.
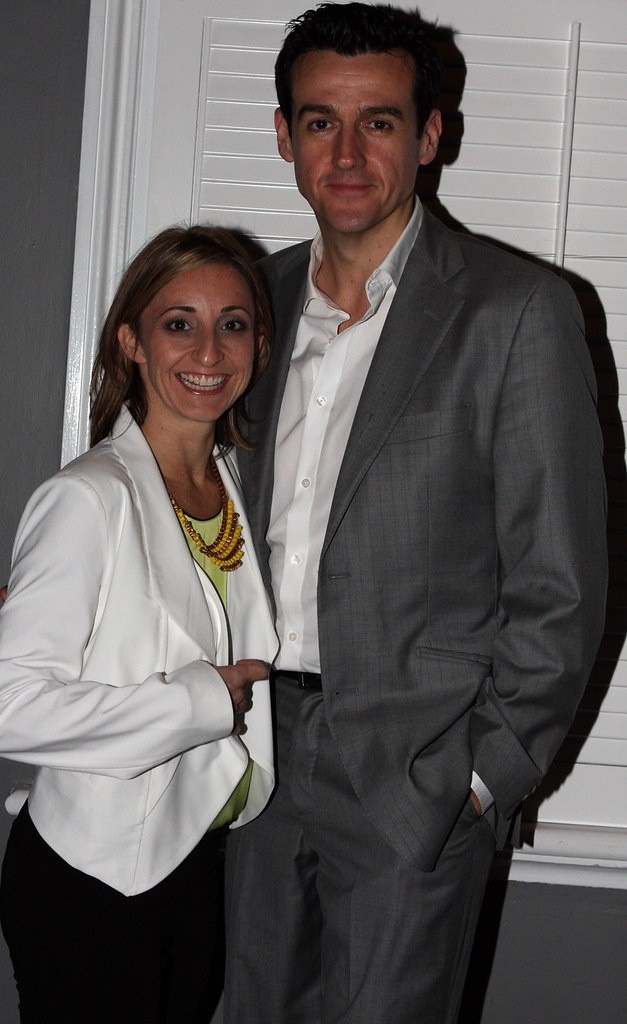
[269,670,322,690]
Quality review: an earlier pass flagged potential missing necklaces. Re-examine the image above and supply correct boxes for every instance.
[165,454,245,572]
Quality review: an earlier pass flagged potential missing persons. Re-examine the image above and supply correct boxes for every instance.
[0,226,279,1024]
[0,1,609,1024]
